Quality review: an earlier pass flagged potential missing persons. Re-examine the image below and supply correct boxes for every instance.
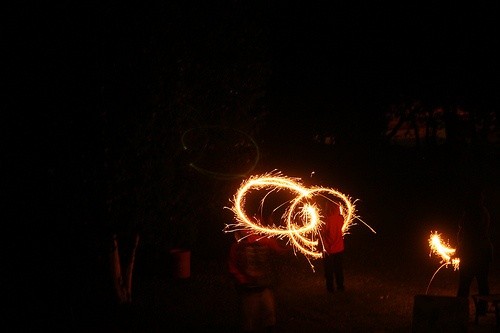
[322,204,345,293]
[458,189,492,317]
[225,214,290,331]
